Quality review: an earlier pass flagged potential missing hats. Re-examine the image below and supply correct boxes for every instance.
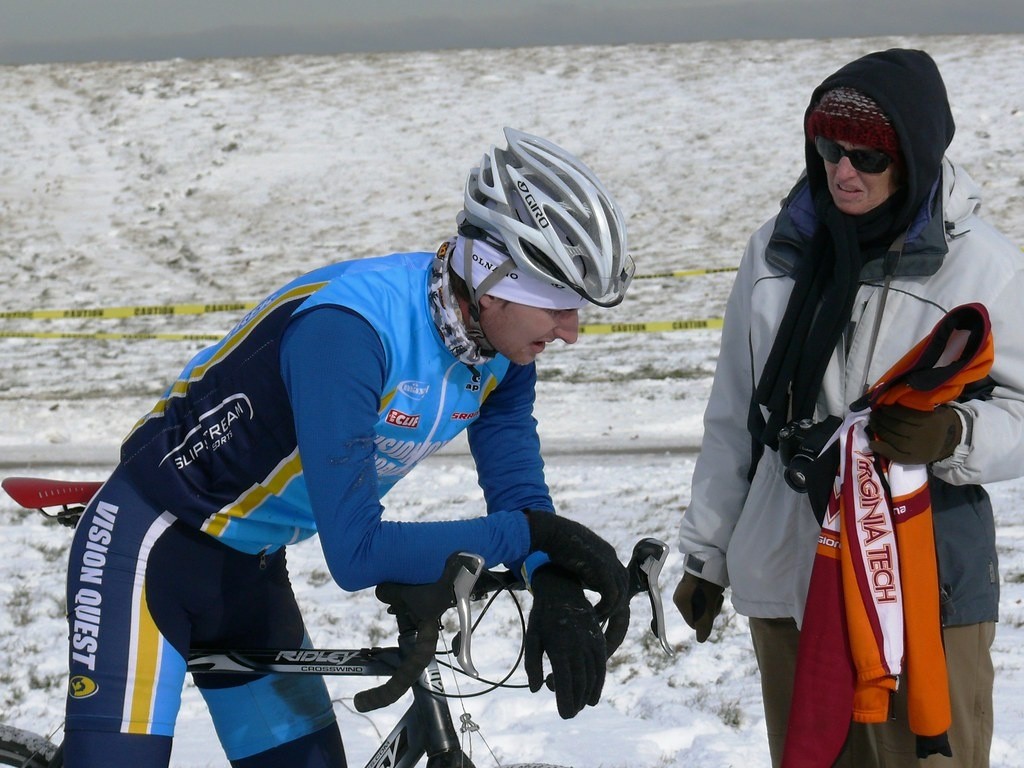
[807,88,901,162]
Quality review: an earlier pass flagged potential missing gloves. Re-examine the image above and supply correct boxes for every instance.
[521,549,607,719]
[674,571,724,643]
[522,505,629,622]
[868,402,964,466]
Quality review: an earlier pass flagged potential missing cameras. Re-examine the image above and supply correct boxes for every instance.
[776,414,846,494]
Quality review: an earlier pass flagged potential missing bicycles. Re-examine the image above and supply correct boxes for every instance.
[0,472,674,768]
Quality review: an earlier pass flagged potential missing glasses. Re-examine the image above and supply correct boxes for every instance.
[813,135,901,174]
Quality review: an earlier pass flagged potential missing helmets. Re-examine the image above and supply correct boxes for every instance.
[463,127,636,308]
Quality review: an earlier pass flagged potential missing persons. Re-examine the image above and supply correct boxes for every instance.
[673,48,1024,768]
[63,126,628,768]
